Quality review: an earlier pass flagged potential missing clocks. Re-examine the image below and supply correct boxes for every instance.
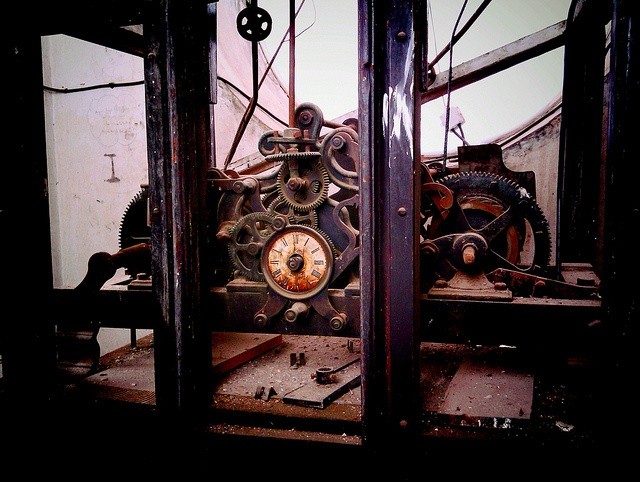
[259,225,334,300]
